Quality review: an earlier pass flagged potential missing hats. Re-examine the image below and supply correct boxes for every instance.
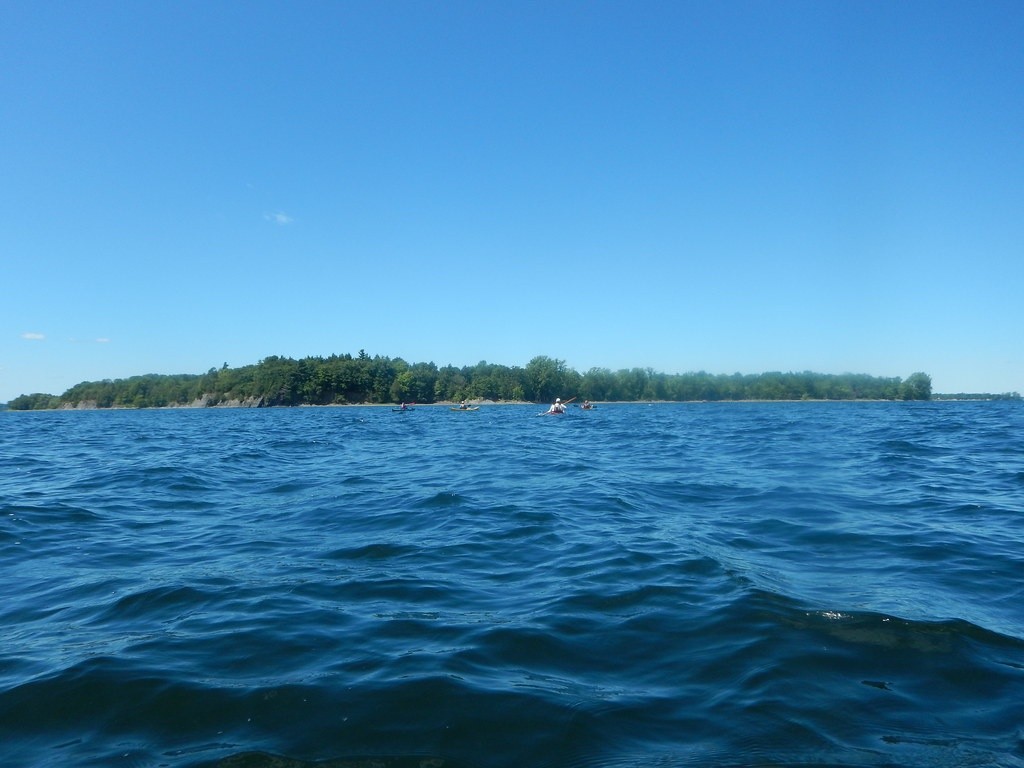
[556,398,561,402]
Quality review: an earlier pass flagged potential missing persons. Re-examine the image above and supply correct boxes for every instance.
[460,401,467,409]
[402,402,407,409]
[548,398,567,412]
[584,400,589,406]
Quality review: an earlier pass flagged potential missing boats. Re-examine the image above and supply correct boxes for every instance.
[551,411,563,414]
[451,405,480,411]
[392,408,415,411]
[581,404,593,409]
